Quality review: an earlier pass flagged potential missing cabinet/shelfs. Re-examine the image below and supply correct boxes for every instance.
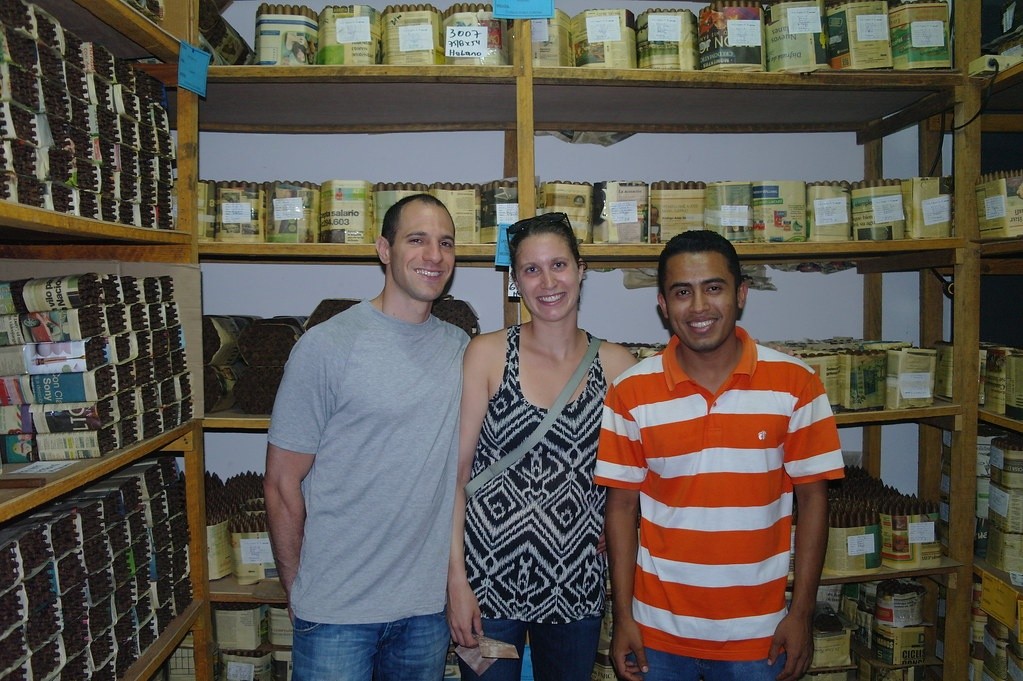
[0,0,218,681]
[914,0,1023,681]
[198,2,969,681]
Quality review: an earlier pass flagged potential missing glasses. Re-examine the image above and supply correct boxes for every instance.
[506,211,575,257]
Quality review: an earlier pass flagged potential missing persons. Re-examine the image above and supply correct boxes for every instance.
[263,193,471,681]
[593,230,846,681]
[447,212,638,681]
